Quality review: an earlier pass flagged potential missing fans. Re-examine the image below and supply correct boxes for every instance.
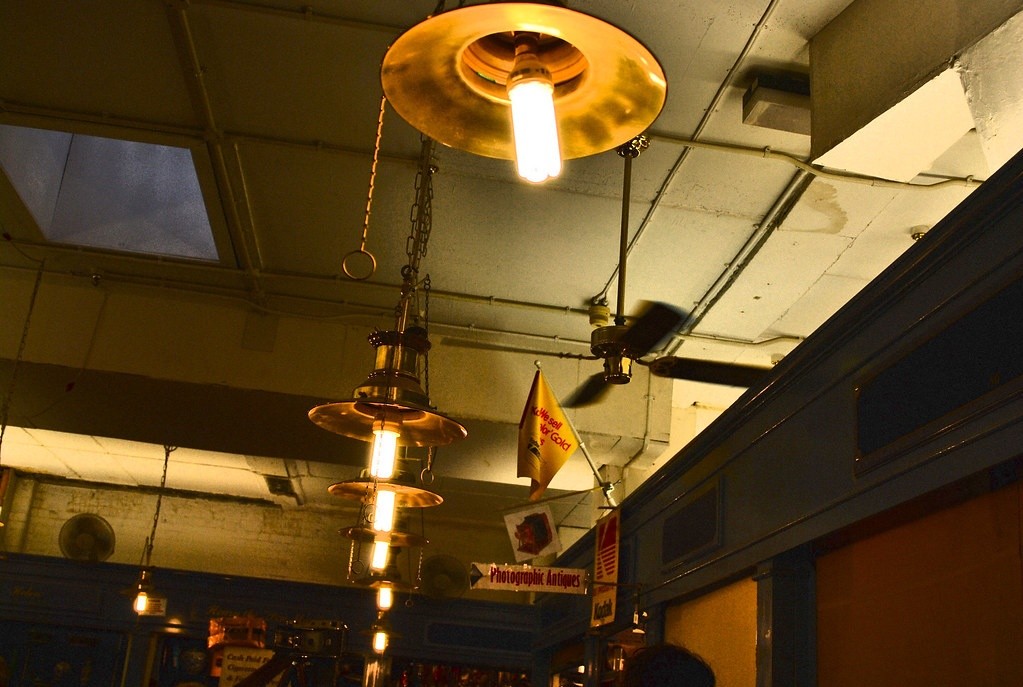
[417,554,468,599]
[440,128,772,409]
[57,513,115,561]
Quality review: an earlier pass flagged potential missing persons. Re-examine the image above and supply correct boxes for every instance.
[623,642,715,687]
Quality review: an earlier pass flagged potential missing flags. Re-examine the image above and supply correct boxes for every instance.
[516,369,578,503]
[501,505,562,564]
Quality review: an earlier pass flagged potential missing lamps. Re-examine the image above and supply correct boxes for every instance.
[307,137,468,483]
[358,614,404,653]
[351,548,416,612]
[336,511,421,573]
[327,441,444,534]
[376,0,668,188]
